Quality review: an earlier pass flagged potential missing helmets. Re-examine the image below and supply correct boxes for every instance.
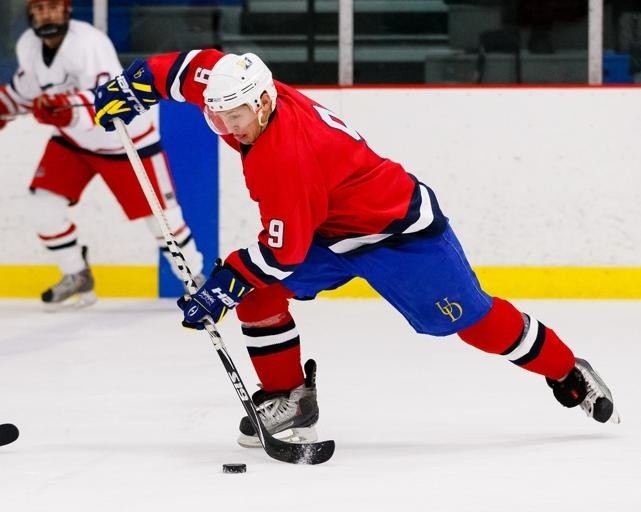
[203,52,277,136]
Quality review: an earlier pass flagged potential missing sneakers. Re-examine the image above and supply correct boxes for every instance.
[548,357,612,423]
[42,268,93,301]
[240,384,318,438]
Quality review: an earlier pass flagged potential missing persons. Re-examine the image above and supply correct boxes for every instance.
[0,0,208,305]
[94,48,613,438]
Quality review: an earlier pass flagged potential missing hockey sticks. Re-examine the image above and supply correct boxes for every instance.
[112,118,335,464]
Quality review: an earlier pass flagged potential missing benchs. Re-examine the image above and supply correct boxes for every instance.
[217,0,468,85]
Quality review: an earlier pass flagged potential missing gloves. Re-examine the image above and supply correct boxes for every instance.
[178,258,255,329]
[33,90,95,125]
[94,59,159,131]
